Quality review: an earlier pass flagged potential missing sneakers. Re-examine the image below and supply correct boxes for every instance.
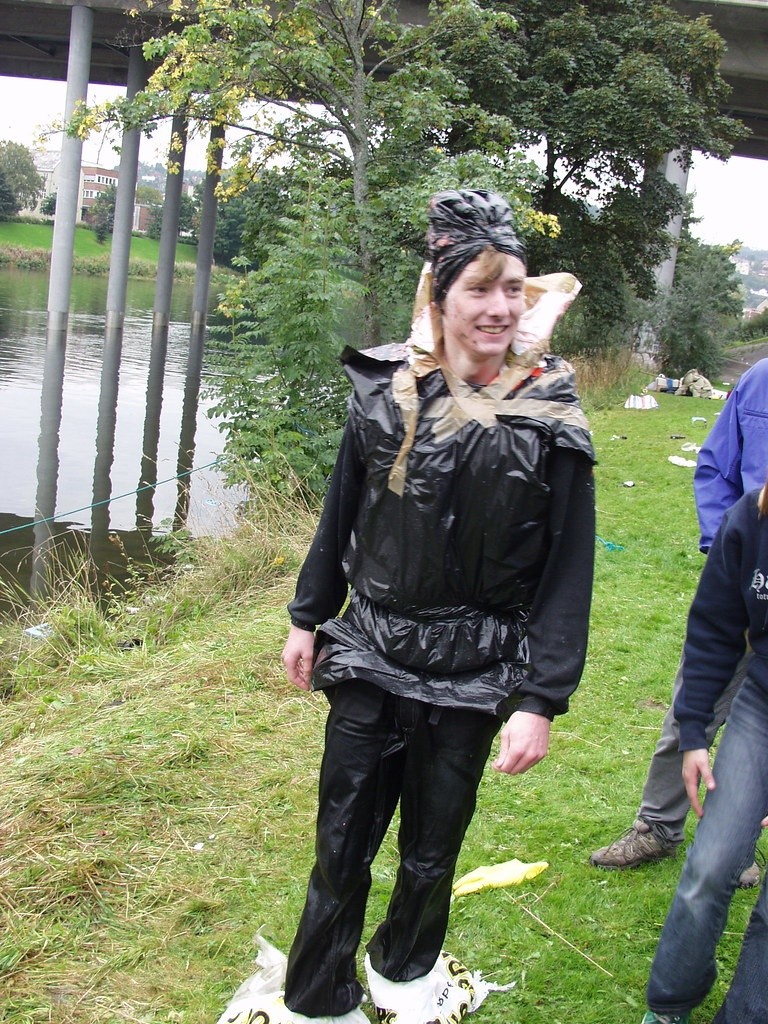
[738,846,766,889]
[589,817,677,871]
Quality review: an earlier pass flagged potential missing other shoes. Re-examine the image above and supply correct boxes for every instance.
[641,1009,689,1024]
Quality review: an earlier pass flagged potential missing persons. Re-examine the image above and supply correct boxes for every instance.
[641,484,768,1024]
[218,188,596,1024]
[590,358,768,889]
